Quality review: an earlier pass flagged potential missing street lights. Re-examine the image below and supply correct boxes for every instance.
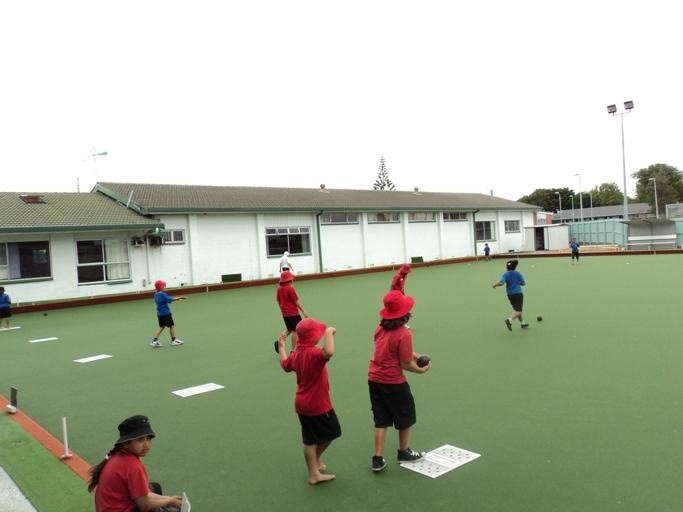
[649,177,659,221]
[606,99,636,250]
[554,170,594,223]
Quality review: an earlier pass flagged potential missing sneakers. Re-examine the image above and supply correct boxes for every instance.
[396,448,425,464]
[151,341,162,347]
[372,456,386,471]
[505,319,512,331]
[521,323,528,327]
[171,340,183,345]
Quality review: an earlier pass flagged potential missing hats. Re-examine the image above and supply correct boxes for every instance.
[379,290,414,319]
[279,271,295,282]
[507,260,518,270]
[296,319,326,345]
[116,415,154,445]
[154,280,166,292]
[400,264,412,274]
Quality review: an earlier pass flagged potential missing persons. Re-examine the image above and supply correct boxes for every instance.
[391,265,412,329]
[0,286,12,329]
[278,318,341,485]
[274,271,307,353]
[87,414,183,512]
[368,290,431,471]
[570,238,580,264]
[150,281,187,347]
[484,243,491,261]
[279,251,293,273]
[493,260,529,331]
